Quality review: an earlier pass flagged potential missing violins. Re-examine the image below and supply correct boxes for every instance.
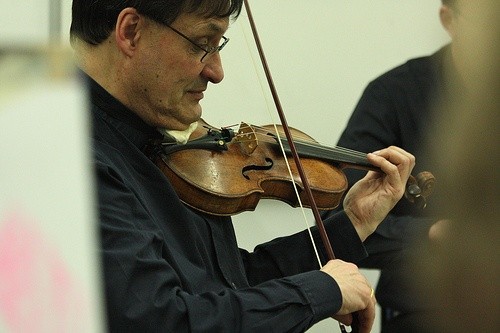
[156,117,437,216]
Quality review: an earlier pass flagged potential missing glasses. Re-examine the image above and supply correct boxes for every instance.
[152,15,230,62]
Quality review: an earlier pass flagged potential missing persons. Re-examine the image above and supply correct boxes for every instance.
[70,0,415,333]
[317,0,500,333]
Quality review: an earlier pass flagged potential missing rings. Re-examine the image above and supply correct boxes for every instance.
[370,289,374,298]
